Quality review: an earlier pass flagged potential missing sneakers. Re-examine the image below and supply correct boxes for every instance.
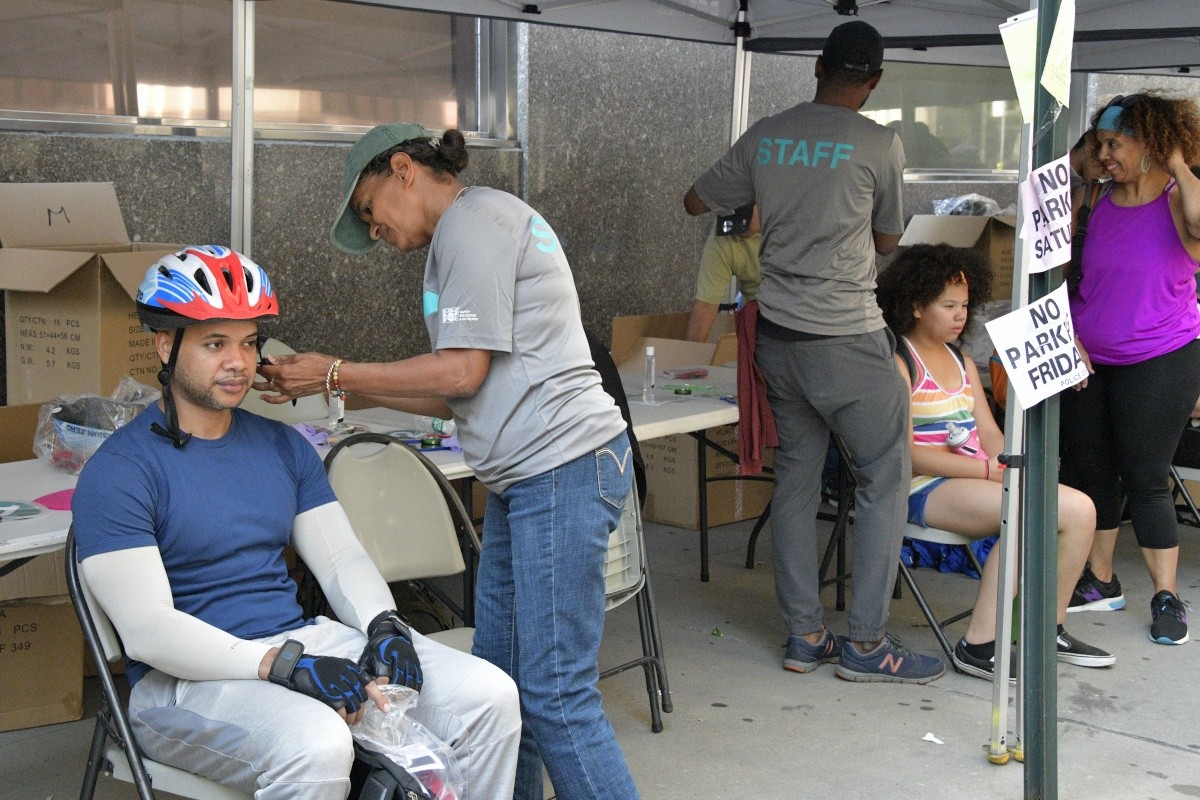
[1066,567,1126,612]
[952,640,1016,683]
[783,627,851,674]
[1149,591,1189,645]
[835,633,945,685]
[1057,631,1116,667]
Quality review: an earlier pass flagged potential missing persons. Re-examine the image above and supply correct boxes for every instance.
[1055,84,1199,646]
[70,244,523,799]
[251,121,645,800]
[687,207,849,496]
[878,240,1115,687]
[683,20,947,685]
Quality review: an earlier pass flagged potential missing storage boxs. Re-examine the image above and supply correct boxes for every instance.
[0,548,85,732]
[608,310,775,531]
[898,214,1017,300]
[0,182,188,463]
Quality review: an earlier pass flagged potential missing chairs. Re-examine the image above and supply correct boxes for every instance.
[818,428,983,674]
[64,333,672,800]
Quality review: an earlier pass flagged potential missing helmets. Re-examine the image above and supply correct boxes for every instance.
[136,244,279,330]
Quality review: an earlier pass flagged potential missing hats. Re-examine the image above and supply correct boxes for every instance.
[330,122,439,253]
[716,199,754,237]
[824,20,885,72]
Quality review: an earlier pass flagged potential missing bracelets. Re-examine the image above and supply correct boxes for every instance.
[332,359,350,401]
[984,460,989,481]
[326,358,345,397]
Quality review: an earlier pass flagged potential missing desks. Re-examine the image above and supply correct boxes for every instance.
[0,364,740,736]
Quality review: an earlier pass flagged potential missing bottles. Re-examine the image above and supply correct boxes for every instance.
[414,414,457,437]
[642,346,656,403]
[328,390,345,427]
[946,422,989,460]
